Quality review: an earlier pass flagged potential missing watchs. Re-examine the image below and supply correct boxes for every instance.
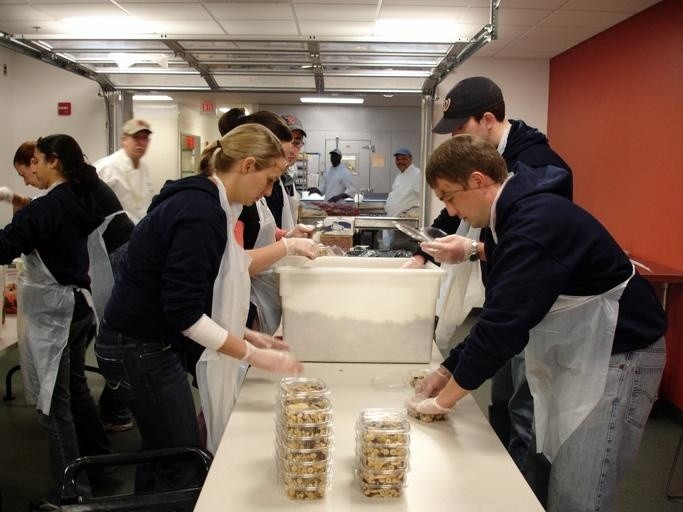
[467,240,479,262]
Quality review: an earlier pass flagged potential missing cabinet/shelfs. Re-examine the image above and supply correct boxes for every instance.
[297,152,307,192]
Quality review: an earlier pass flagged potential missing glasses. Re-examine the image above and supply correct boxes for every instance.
[291,140,305,146]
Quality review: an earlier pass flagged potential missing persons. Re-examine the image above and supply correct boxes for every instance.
[384,147,420,250]
[95,123,304,512]
[0,134,120,512]
[93,119,154,227]
[177,108,307,390]
[405,76,572,488]
[309,149,356,202]
[0,141,134,433]
[407,134,668,512]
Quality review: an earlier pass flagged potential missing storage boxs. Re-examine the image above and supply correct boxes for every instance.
[276,258,446,363]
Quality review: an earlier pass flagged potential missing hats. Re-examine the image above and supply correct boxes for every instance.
[431,76,505,134]
[393,148,412,158]
[282,114,307,138]
[121,118,153,134]
[329,149,342,157]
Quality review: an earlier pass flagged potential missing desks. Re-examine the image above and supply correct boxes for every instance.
[0,310,101,399]
[624,251,683,311]
[194,320,547,512]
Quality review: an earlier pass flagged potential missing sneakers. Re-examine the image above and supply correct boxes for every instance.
[100,411,133,432]
